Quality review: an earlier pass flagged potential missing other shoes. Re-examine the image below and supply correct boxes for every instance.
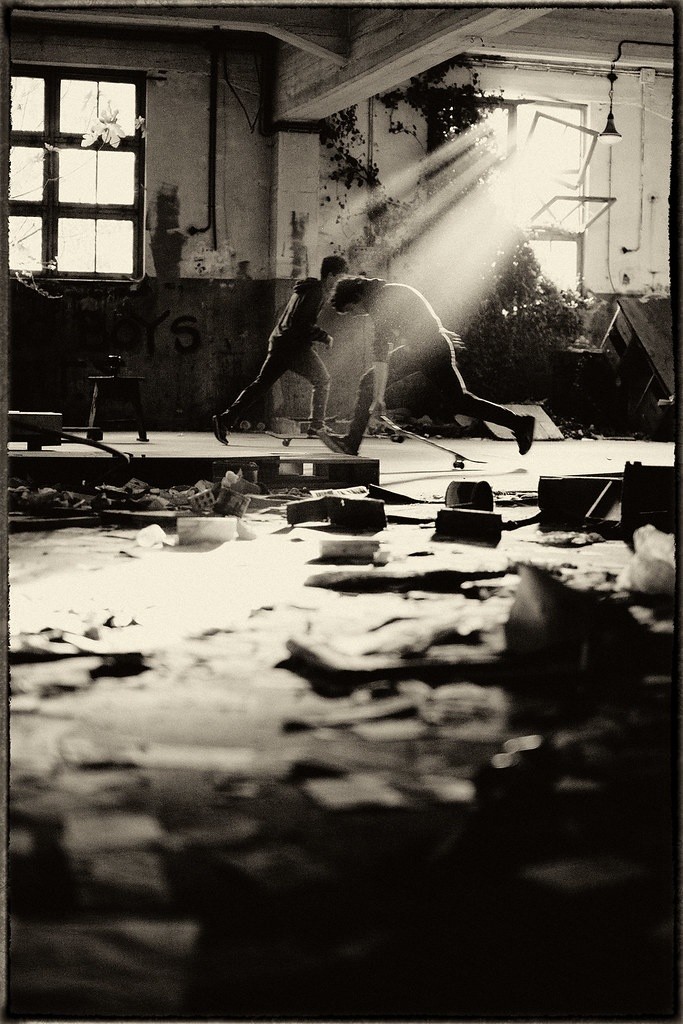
[510,414,535,455]
[321,434,359,456]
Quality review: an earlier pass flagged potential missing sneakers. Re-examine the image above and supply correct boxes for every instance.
[307,424,336,435]
[212,415,229,446]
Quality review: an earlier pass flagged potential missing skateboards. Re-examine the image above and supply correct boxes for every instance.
[372,414,488,470]
[263,430,347,447]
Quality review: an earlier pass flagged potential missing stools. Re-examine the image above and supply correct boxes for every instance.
[88,376,150,442]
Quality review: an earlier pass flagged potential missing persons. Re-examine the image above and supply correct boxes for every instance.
[212,255,348,445]
[318,272,537,455]
